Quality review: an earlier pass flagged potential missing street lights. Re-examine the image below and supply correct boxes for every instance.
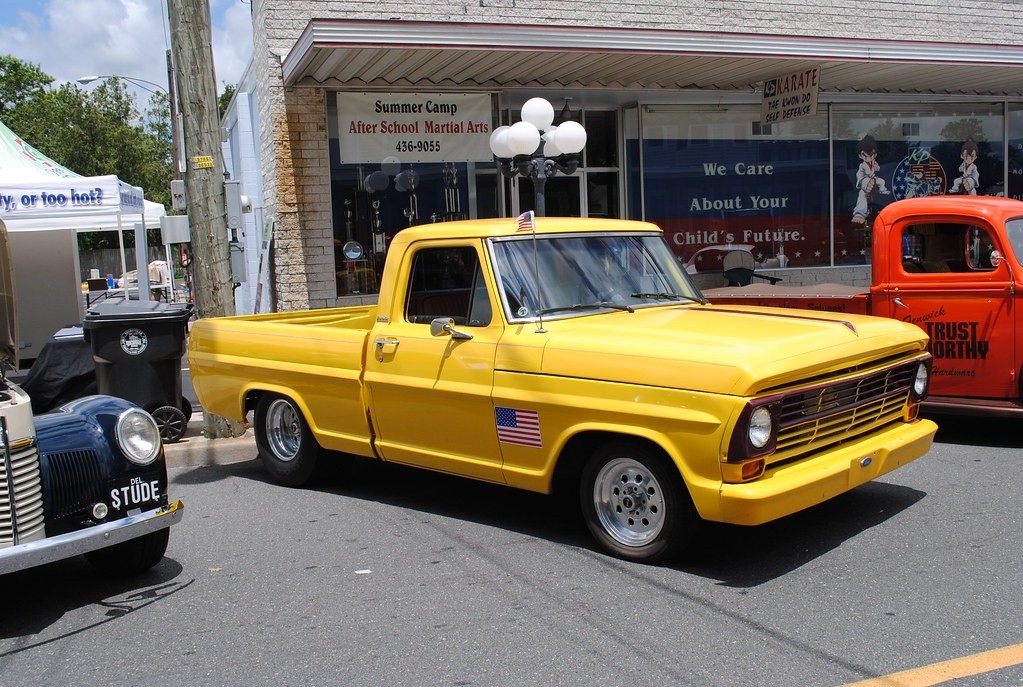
[77,48,182,181]
[489,95,587,216]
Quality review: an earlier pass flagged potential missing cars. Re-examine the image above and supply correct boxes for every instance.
[0,352,185,616]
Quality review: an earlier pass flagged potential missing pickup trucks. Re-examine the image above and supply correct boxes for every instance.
[187,210,939,563]
[695,195,1022,420]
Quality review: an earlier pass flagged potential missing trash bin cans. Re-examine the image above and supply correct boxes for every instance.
[83,298,196,444]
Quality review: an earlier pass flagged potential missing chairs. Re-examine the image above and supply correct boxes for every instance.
[921,260,952,272]
[417,292,469,317]
[722,250,783,288]
[903,262,922,273]
